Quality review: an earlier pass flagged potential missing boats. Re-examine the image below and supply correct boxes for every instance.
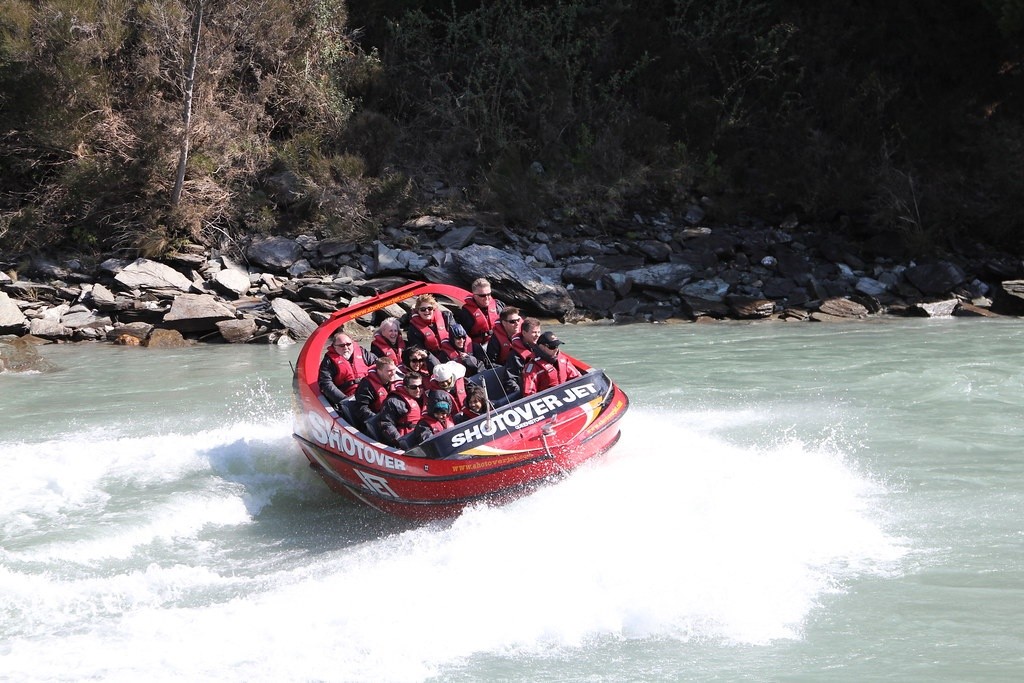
[290,281,634,527]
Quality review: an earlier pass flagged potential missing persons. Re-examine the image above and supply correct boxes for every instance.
[318,278,582,451]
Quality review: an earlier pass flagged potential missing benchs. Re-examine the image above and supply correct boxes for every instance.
[338,395,360,428]
[468,366,516,406]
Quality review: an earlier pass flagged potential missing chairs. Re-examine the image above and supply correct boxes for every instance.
[364,407,381,442]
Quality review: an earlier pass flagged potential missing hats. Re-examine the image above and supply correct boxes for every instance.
[429,363,455,382]
[537,331,565,347]
[432,401,449,413]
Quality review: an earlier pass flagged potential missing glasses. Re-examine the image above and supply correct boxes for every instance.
[419,307,433,311]
[335,343,351,347]
[476,293,492,297]
[407,384,423,389]
[505,318,520,323]
[544,344,556,350]
[455,336,466,340]
[410,359,425,363]
